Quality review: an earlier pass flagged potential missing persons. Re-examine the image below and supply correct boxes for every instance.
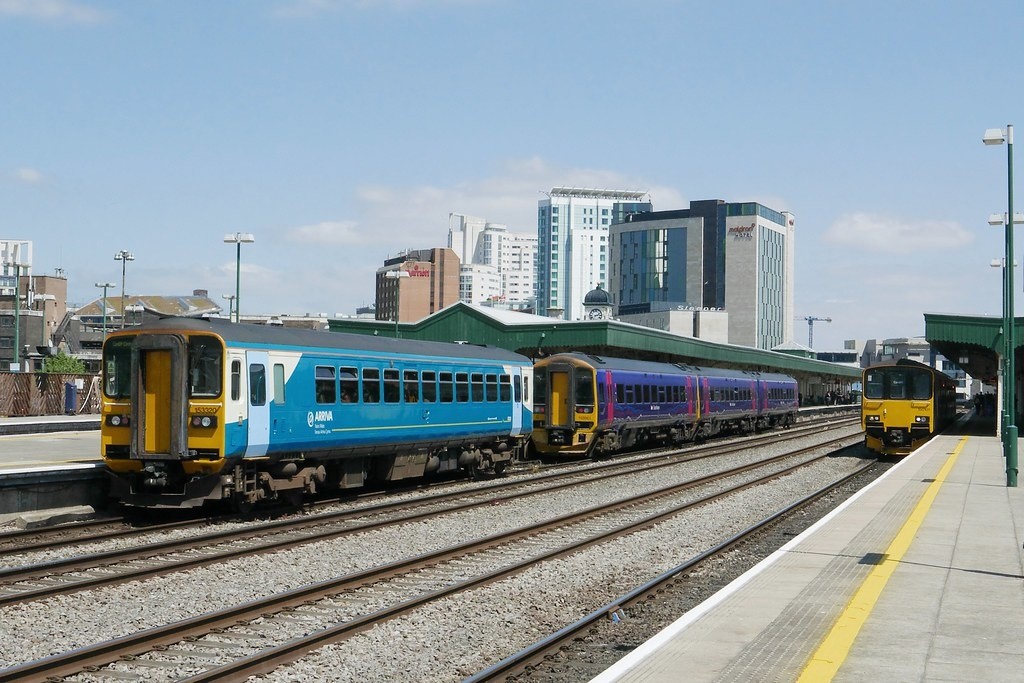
[825,388,857,406]
[798,391,803,408]
[974,391,997,409]
[363,386,374,402]
[423,395,430,402]
[404,374,419,403]
[340,386,358,403]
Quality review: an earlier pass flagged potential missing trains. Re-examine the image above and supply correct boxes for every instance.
[858,362,961,459]
[84,315,801,524]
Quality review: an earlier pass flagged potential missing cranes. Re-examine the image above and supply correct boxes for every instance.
[793,316,831,349]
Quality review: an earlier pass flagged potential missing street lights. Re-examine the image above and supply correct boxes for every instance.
[981,124,1019,488]
[223,233,255,323]
[989,256,1017,487]
[987,211,1019,487]
[5,260,31,371]
[95,282,116,348]
[223,294,235,321]
[33,293,56,372]
[113,249,135,330]
[387,271,410,339]
